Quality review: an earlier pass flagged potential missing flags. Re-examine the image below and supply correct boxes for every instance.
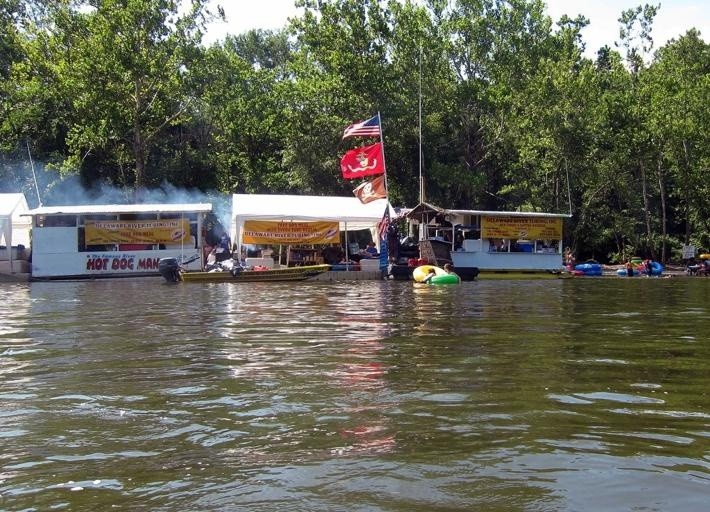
[352,174,387,204]
[377,205,389,242]
[340,141,385,180]
[341,114,381,142]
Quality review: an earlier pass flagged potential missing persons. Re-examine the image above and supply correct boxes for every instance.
[217,232,232,255]
[420,268,436,283]
[564,246,576,272]
[444,263,462,284]
[445,230,479,254]
[322,236,377,272]
[685,257,698,276]
[626,257,639,277]
[644,259,652,277]
[695,258,709,277]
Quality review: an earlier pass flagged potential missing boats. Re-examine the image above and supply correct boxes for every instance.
[616,261,663,276]
[413,265,459,284]
[574,264,603,276]
[157,253,333,283]
